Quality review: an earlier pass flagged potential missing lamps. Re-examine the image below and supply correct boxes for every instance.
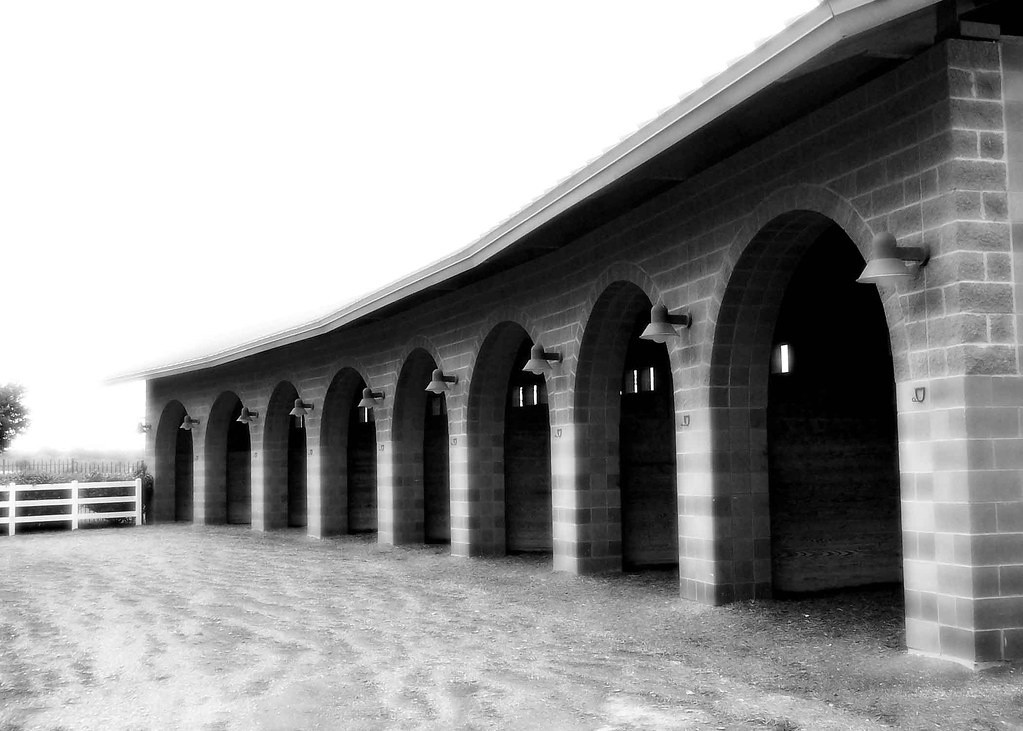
[639,302,693,344]
[522,343,564,375]
[236,407,259,424]
[288,399,315,418]
[854,232,930,286]
[425,368,460,395]
[358,388,385,411]
[136,422,151,434]
[179,415,200,430]
